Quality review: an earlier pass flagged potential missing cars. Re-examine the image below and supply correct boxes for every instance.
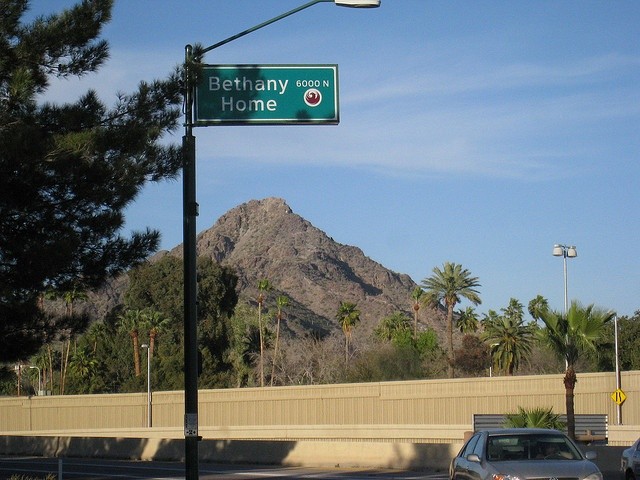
[449,428,603,480]
[620,437,640,480]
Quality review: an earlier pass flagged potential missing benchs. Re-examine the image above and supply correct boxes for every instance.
[463,415,607,448]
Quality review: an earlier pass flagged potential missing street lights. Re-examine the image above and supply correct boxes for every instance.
[552,242,577,369]
[182,0,382,479]
[141,344,152,427]
[29,366,41,391]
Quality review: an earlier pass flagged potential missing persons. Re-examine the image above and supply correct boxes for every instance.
[535,443,573,459]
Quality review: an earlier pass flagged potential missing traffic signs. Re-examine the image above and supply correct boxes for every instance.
[190,64,340,126]
